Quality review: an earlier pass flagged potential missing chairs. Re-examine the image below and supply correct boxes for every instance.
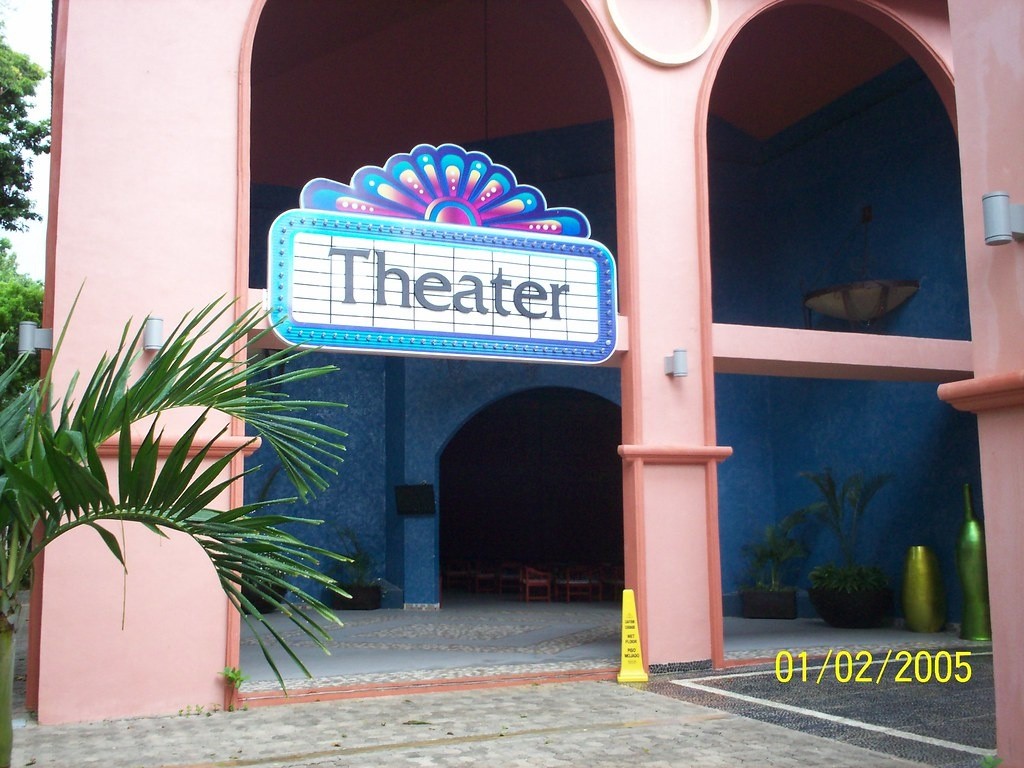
[441,559,624,605]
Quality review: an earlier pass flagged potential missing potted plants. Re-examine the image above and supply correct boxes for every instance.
[796,463,896,631]
[319,521,385,606]
[729,508,811,618]
